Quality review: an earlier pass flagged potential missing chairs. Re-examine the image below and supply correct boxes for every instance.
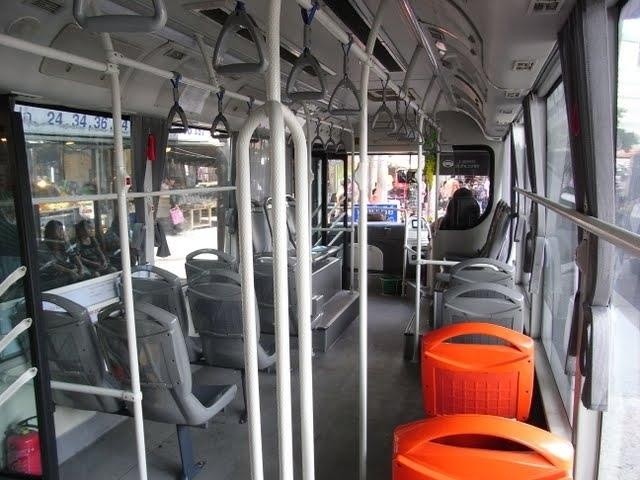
[393,200,574,480]
[12,193,342,480]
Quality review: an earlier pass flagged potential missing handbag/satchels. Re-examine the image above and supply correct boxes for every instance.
[168,205,187,225]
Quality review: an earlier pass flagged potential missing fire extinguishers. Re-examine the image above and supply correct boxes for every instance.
[4,416,43,480]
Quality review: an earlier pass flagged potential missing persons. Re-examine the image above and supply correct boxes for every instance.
[0,192,136,282]
[327,160,489,241]
[157,176,184,233]
